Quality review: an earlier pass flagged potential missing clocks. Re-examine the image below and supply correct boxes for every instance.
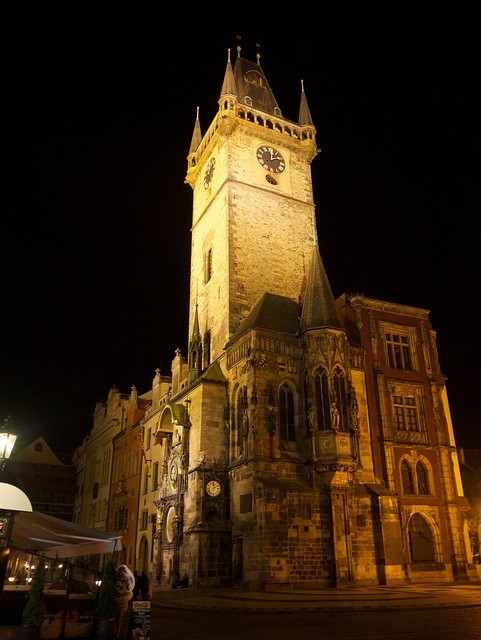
[255,144,287,174]
[205,480,220,498]
[202,157,216,190]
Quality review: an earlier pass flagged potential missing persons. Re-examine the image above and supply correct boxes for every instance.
[140,572,149,600]
[132,570,140,598]
